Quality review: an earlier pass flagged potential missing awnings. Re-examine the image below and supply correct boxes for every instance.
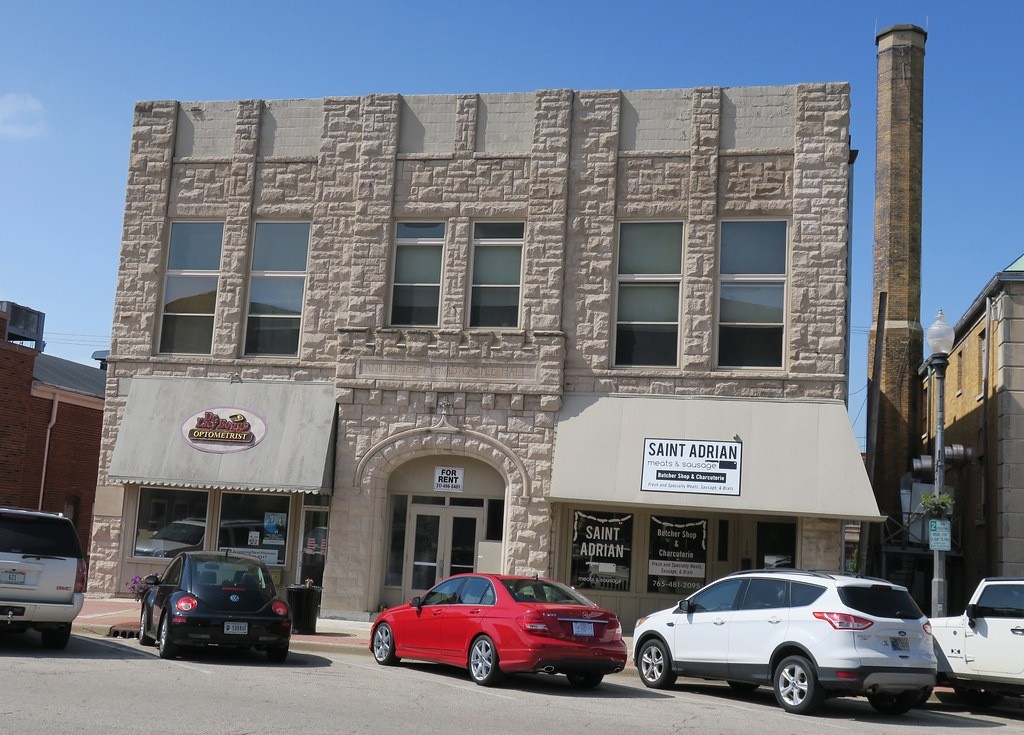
[104,374,338,495]
[545,391,890,524]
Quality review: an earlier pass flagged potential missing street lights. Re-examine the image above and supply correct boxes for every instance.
[927,310,957,617]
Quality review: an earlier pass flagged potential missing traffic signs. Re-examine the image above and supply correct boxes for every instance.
[929,519,952,552]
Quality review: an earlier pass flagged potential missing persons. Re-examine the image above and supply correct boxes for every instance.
[266,513,285,536]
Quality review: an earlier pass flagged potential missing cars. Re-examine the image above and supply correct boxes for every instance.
[367,573,628,692]
[300,525,329,570]
[137,550,292,665]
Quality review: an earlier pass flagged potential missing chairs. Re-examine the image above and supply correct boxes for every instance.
[468,582,488,604]
[200,571,217,585]
[234,571,251,583]
[756,585,779,606]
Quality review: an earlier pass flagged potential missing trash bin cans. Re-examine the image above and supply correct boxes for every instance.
[286,583,321,636]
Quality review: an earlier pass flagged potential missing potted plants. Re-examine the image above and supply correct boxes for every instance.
[919,490,956,520]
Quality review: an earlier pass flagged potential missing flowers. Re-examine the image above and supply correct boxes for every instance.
[126,571,164,603]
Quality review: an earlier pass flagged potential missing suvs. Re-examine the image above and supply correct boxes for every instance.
[631,566,939,717]
[134,515,288,565]
[923,577,1024,709]
[0,506,87,651]
